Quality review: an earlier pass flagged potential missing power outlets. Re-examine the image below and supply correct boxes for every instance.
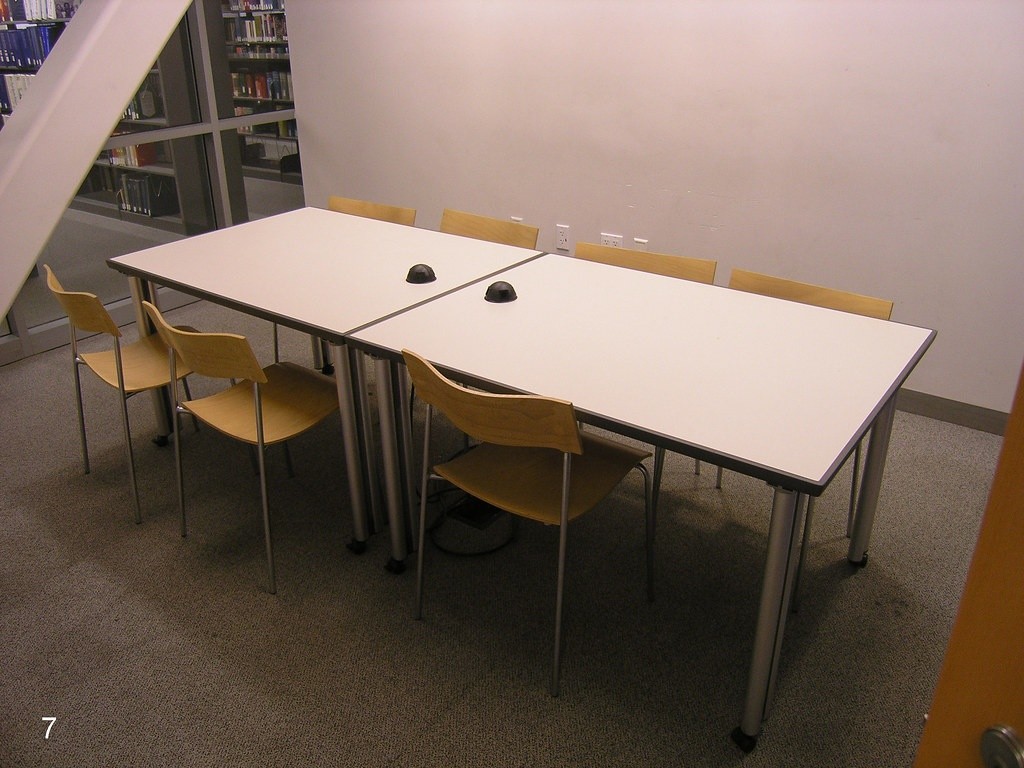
[601,233,626,249]
[555,224,570,250]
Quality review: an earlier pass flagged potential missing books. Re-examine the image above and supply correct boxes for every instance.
[0,74,36,132]
[76,123,181,216]
[118,73,164,120]
[0,0,87,70]
[220,0,298,139]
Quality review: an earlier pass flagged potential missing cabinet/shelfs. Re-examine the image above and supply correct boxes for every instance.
[227,9,302,189]
[0,0,216,238]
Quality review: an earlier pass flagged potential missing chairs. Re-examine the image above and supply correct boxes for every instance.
[43,199,894,698]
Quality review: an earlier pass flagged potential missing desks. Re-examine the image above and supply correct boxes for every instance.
[351,255,939,753]
[103,204,543,551]
